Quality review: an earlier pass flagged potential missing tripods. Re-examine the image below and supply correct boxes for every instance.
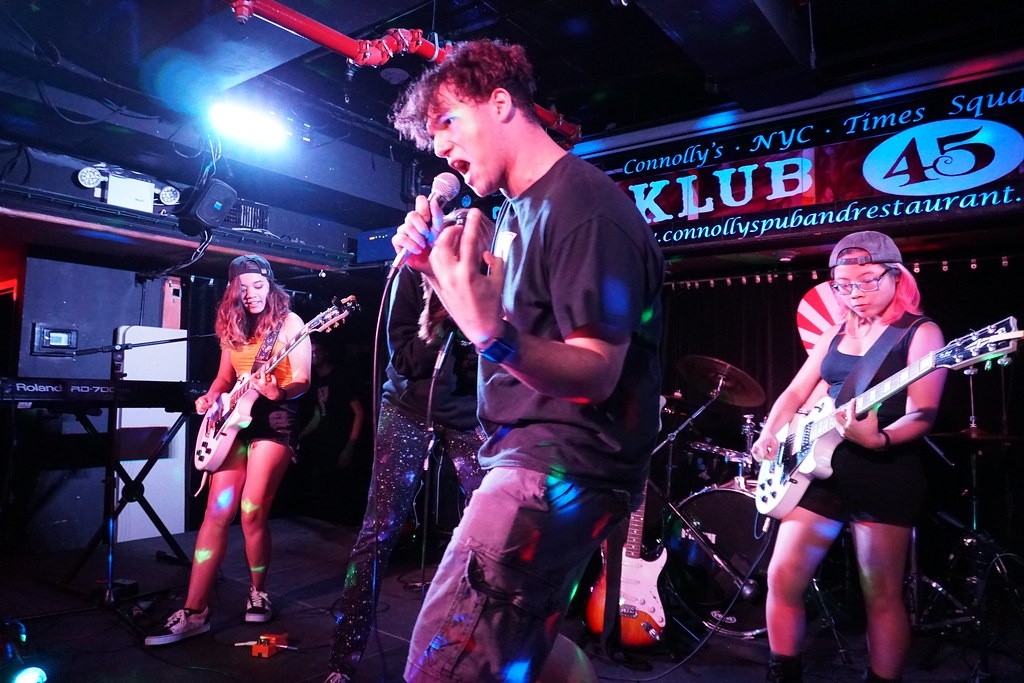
[902,439,1024,643]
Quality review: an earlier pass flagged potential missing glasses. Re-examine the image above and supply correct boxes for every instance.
[830,268,894,296]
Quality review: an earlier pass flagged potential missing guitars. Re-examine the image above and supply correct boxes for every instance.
[754,312,1024,521]
[584,395,669,648]
[193,293,359,473]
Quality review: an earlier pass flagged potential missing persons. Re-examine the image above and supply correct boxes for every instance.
[752,230,949,683]
[295,342,364,517]
[393,36,666,683]
[145,255,312,647]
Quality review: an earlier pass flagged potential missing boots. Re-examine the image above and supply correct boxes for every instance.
[861,668,903,683]
[763,650,804,683]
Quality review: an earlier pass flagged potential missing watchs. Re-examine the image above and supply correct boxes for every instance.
[474,322,519,363]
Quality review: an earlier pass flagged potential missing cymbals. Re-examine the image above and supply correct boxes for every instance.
[680,353,766,408]
[927,427,1018,444]
[661,394,693,416]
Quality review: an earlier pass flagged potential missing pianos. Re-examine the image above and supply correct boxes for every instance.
[0,376,213,605]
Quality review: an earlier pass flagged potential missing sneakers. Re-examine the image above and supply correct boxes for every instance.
[144,604,211,646]
[245,585,272,623]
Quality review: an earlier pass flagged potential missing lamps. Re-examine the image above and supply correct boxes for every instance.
[78,167,180,214]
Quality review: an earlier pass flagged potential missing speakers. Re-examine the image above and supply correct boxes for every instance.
[190,180,238,228]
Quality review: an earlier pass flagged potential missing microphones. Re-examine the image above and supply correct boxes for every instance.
[385,171,460,280]
[733,575,762,602]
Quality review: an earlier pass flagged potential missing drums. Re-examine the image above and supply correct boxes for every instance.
[676,439,753,493]
[919,511,1003,615]
[660,477,824,637]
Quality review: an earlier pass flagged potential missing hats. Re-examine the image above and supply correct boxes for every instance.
[828,231,903,267]
[227,254,275,282]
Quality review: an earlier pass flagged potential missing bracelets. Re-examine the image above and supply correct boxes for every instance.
[277,388,287,402]
[880,431,890,450]
